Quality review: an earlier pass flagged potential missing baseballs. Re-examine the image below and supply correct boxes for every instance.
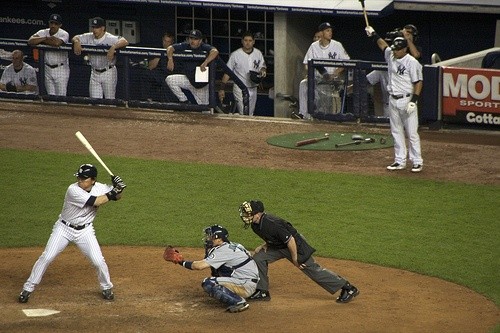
[324,132,329,137]
[341,133,345,137]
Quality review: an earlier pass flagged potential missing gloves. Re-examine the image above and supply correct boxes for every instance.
[365,25,375,36]
[111,175,121,187]
[105,182,126,201]
[405,102,416,114]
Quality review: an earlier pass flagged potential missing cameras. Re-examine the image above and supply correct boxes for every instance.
[384,30,404,46]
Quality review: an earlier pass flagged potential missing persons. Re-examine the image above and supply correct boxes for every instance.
[364,26,424,173]
[238,200,360,304]
[291,23,350,120]
[17,163,127,304]
[0,50,39,95]
[218,32,267,116]
[400,25,421,59]
[163,224,261,313]
[347,70,388,117]
[71,17,129,107]
[129,42,168,101]
[314,29,320,42]
[163,32,174,49]
[27,14,71,104]
[165,30,219,114]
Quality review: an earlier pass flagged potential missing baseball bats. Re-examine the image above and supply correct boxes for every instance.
[75,130,127,189]
[294,136,329,146]
[335,138,375,147]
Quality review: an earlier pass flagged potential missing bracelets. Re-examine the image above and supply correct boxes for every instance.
[410,93,419,104]
[105,190,116,201]
[372,32,381,43]
[185,260,194,270]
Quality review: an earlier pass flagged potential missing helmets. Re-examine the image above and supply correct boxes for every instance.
[73,164,97,178]
[202,225,229,248]
[389,38,407,51]
[239,200,264,229]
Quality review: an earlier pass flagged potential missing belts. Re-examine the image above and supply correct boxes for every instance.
[92,66,113,72]
[45,63,63,68]
[391,95,410,99]
[62,221,90,230]
[251,279,258,282]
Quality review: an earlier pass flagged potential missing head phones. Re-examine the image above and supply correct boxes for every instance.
[411,29,418,37]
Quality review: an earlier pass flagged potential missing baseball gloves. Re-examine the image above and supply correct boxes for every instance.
[163,245,185,264]
[250,68,266,84]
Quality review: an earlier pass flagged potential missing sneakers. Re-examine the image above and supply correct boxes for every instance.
[411,164,422,172]
[20,290,30,302]
[102,289,114,299]
[386,162,406,170]
[336,286,360,303]
[291,111,303,119]
[319,22,331,30]
[226,302,249,312]
[246,289,271,302]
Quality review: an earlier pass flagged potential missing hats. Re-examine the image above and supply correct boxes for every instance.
[187,30,202,39]
[49,14,61,23]
[250,71,263,82]
[88,17,105,27]
